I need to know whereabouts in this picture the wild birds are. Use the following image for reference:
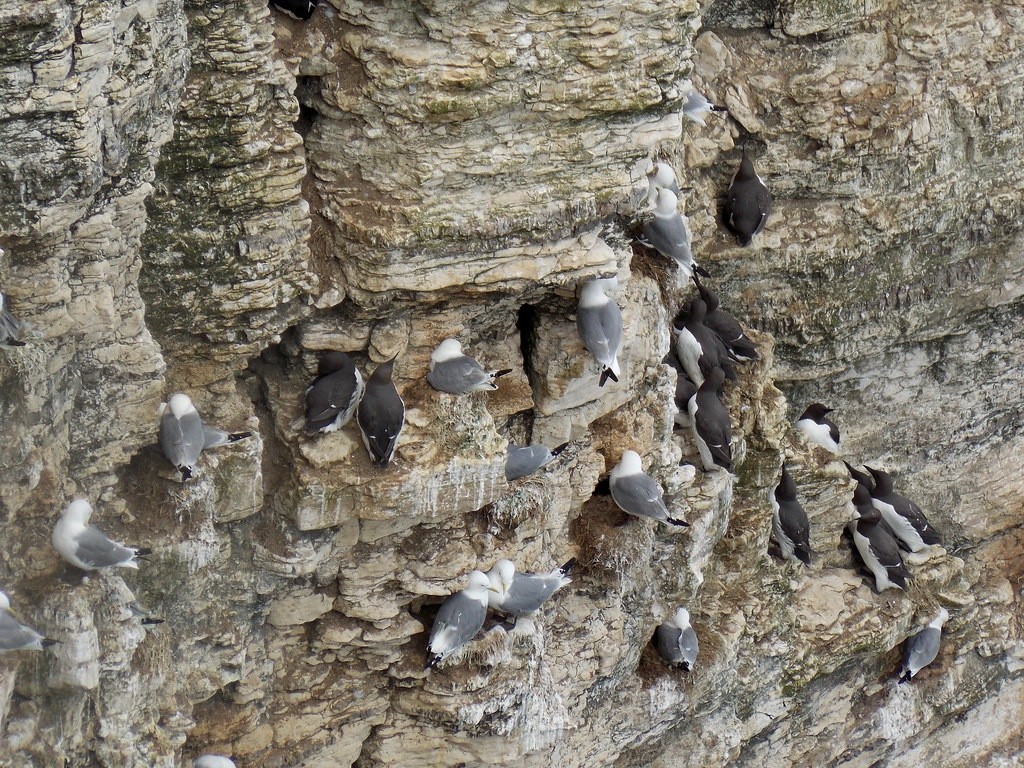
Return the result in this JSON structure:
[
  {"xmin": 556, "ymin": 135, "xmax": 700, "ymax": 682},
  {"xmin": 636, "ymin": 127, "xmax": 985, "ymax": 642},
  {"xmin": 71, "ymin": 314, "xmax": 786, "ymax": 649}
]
[{"xmin": 0, "ymin": 1, "xmax": 951, "ymax": 768}]
